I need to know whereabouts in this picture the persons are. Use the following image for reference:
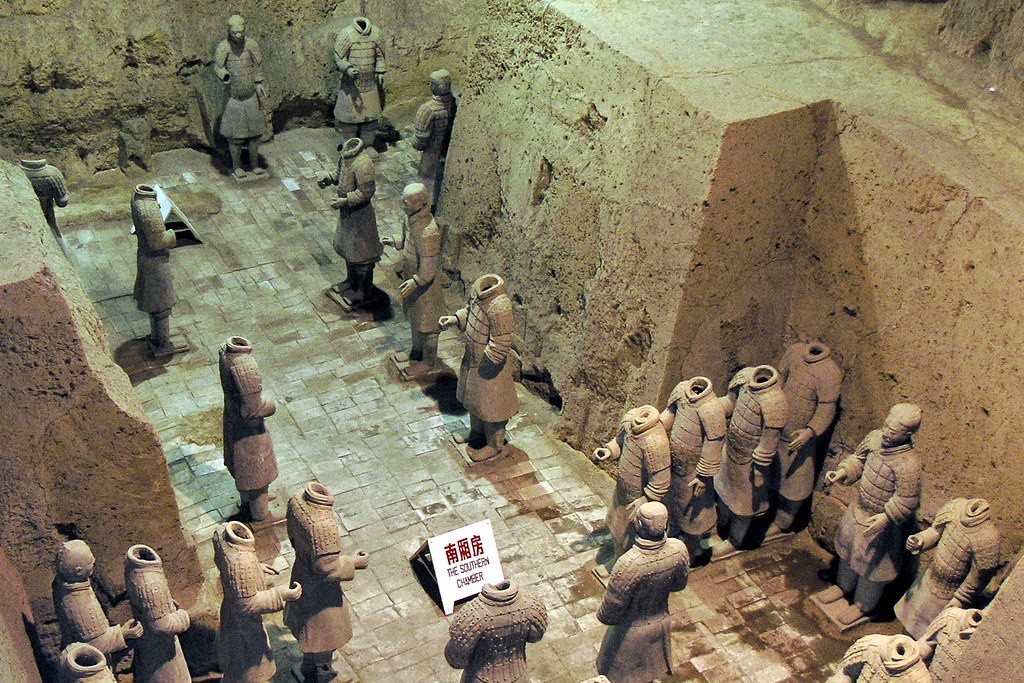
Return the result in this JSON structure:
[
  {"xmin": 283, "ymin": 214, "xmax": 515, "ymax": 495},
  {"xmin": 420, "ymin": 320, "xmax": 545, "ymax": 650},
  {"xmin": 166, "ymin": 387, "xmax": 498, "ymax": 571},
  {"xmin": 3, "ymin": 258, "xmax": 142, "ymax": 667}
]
[
  {"xmin": 22, "ymin": 16, "xmax": 1000, "ymax": 682},
  {"xmin": 377, "ymin": 182, "xmax": 448, "ymax": 376}
]
